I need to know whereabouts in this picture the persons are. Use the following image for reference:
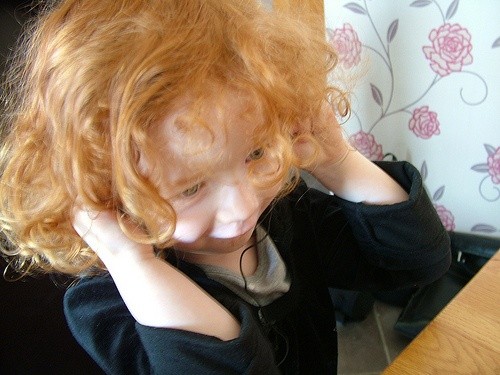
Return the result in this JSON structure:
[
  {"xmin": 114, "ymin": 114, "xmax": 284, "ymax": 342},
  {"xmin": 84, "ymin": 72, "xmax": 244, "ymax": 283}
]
[{"xmin": 0, "ymin": 0, "xmax": 452, "ymax": 375}]
